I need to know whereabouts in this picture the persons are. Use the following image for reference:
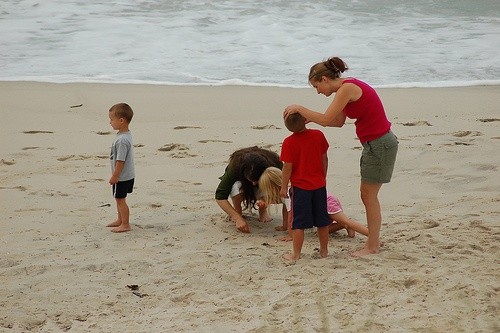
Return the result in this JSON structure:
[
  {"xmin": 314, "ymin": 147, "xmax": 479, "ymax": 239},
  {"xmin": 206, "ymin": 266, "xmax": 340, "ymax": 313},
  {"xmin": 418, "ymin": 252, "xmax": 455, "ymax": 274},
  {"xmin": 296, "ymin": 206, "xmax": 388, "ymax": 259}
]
[
  {"xmin": 214, "ymin": 146, "xmax": 284, "ymax": 233},
  {"xmin": 279, "ymin": 113, "xmax": 331, "ymax": 262},
  {"xmin": 259, "ymin": 166, "xmax": 385, "ymax": 248},
  {"xmin": 283, "ymin": 57, "xmax": 399, "ymax": 257},
  {"xmin": 105, "ymin": 102, "xmax": 136, "ymax": 234}
]
